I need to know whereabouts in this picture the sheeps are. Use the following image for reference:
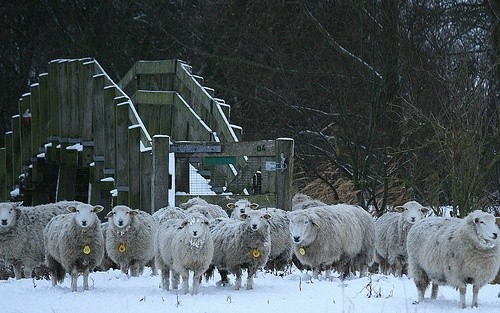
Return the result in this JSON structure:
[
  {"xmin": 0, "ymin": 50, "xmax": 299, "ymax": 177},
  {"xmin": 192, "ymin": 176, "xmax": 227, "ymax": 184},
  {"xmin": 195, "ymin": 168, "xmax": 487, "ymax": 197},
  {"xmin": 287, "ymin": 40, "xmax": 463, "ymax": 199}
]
[
  {"xmin": 226, "ymin": 198, "xmax": 294, "ymax": 271},
  {"xmin": 151, "ymin": 206, "xmax": 187, "ymax": 275},
  {"xmin": 104, "ymin": 205, "xmax": 157, "ymax": 277},
  {"xmin": 286, "ymin": 204, "xmax": 376, "ymax": 277},
  {"xmin": 92, "ymin": 220, "xmax": 120, "ymax": 271},
  {"xmin": 184, "ymin": 204, "xmax": 215, "ymax": 223},
  {"xmin": 43, "ymin": 202, "xmax": 105, "ymax": 291},
  {"xmin": 204, "ymin": 210, "xmax": 272, "ymax": 289},
  {"xmin": 406, "ymin": 209, "xmax": 500, "ymax": 308},
  {"xmin": 154, "ymin": 211, "xmax": 214, "ymax": 295},
  {"xmin": 292, "ymin": 194, "xmax": 328, "ymax": 210},
  {"xmin": 179, "ymin": 197, "xmax": 229, "ymax": 219},
  {"xmin": 375, "ymin": 200, "xmax": 429, "ymax": 277},
  {"xmin": 0, "ymin": 199, "xmax": 84, "ymax": 279}
]
